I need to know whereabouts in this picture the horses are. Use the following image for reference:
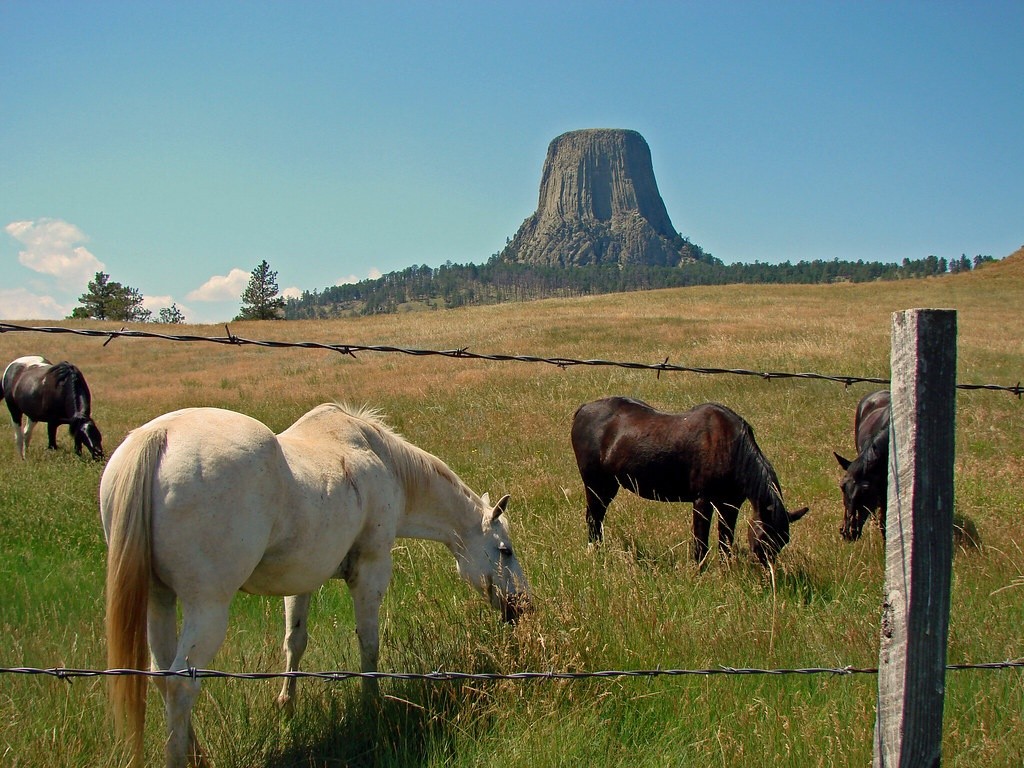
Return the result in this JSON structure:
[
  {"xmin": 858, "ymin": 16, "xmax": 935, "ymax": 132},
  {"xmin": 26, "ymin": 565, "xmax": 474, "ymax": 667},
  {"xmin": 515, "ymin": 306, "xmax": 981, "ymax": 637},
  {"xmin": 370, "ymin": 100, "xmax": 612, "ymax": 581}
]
[
  {"xmin": 97, "ymin": 398, "xmax": 533, "ymax": 768},
  {"xmin": 570, "ymin": 388, "xmax": 892, "ymax": 577},
  {"xmin": 0, "ymin": 354, "xmax": 105, "ymax": 464}
]
